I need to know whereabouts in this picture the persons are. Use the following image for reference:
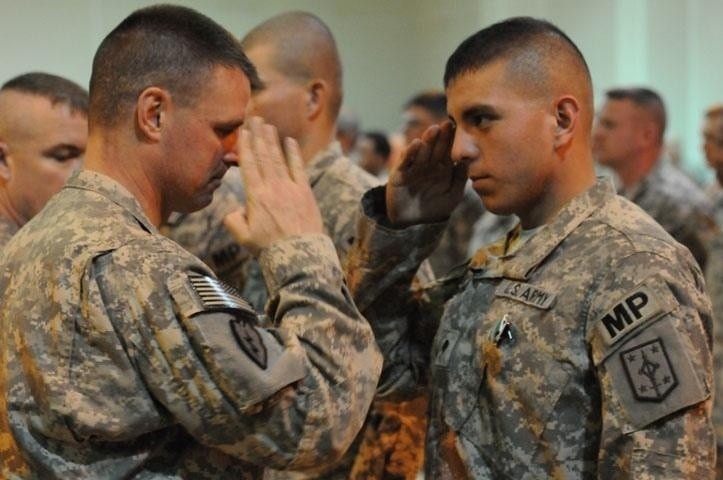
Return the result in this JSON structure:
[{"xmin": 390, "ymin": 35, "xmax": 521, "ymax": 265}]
[
  {"xmin": 336, "ymin": 82, "xmax": 722, "ymax": 480},
  {"xmin": 168, "ymin": 9, "xmax": 386, "ymax": 320},
  {"xmin": 0, "ymin": 70, "xmax": 89, "ymax": 480},
  {"xmin": 0, "ymin": 4, "xmax": 385, "ymax": 480},
  {"xmin": 347, "ymin": 17, "xmax": 716, "ymax": 480}
]
